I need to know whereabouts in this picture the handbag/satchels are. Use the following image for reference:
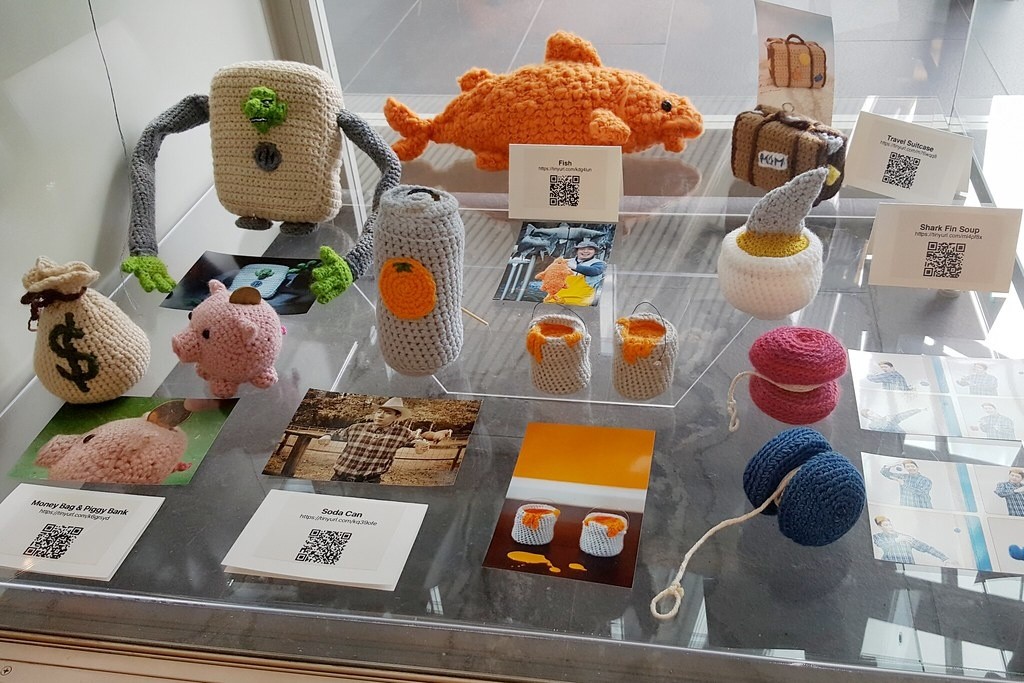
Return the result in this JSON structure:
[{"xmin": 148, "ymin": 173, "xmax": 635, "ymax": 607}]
[
  {"xmin": 731, "ymin": 104, "xmax": 848, "ymax": 209},
  {"xmin": 764, "ymin": 34, "xmax": 826, "ymax": 88}
]
